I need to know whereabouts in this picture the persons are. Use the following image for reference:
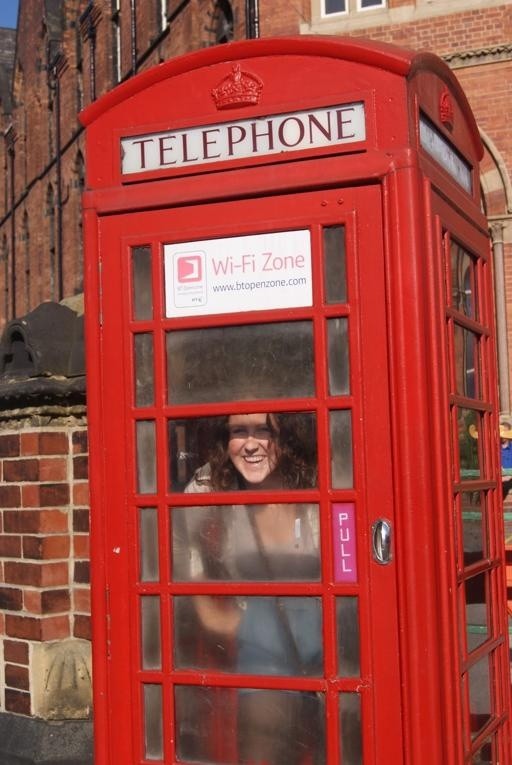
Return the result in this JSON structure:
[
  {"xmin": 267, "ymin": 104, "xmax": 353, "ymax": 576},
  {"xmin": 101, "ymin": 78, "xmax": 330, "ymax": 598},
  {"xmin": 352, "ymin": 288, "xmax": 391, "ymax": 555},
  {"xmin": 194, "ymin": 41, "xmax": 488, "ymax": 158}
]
[
  {"xmin": 500, "ymin": 422, "xmax": 512, "ymax": 469},
  {"xmin": 169, "ymin": 386, "xmax": 365, "ymax": 765}
]
